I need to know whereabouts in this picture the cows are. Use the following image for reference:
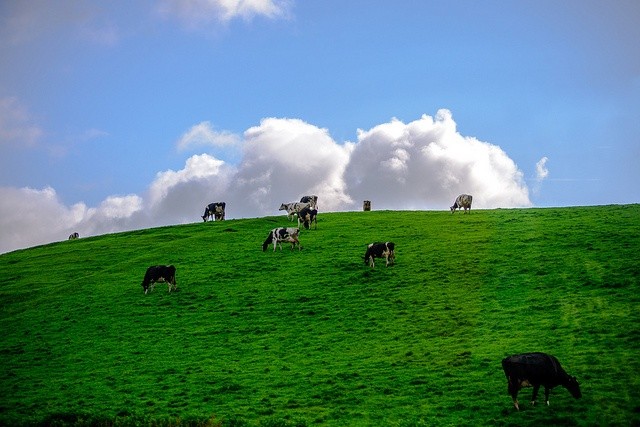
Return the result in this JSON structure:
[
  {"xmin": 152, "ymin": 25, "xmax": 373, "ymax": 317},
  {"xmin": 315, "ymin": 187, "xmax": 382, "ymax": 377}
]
[
  {"xmin": 279, "ymin": 203, "xmax": 310, "ymax": 223},
  {"xmin": 450, "ymin": 194, "xmax": 472, "ymax": 215},
  {"xmin": 263, "ymin": 227, "xmax": 301, "ymax": 252},
  {"xmin": 69, "ymin": 233, "xmax": 78, "ymax": 240},
  {"xmin": 363, "ymin": 201, "xmax": 370, "ymax": 211},
  {"xmin": 300, "ymin": 196, "xmax": 318, "ymax": 208},
  {"xmin": 300, "ymin": 207, "xmax": 317, "ymax": 229},
  {"xmin": 201, "ymin": 202, "xmax": 226, "ymax": 222},
  {"xmin": 141, "ymin": 265, "xmax": 176, "ymax": 294},
  {"xmin": 502, "ymin": 352, "xmax": 582, "ymax": 410},
  {"xmin": 365, "ymin": 242, "xmax": 394, "ymax": 268}
]
